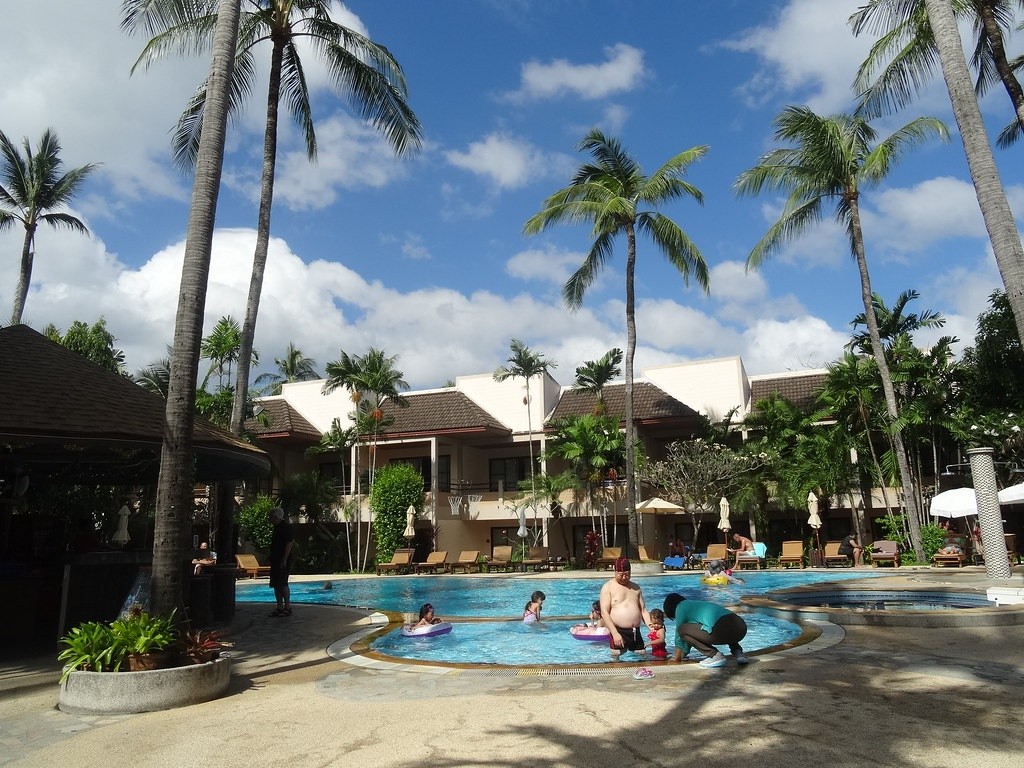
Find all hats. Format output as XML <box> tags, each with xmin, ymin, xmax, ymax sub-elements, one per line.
<box><xmin>614</xmin><ymin>556</ymin><xmax>631</xmax><ymax>572</ymax></box>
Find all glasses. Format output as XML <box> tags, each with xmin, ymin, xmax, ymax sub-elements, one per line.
<box><xmin>591</xmin><ymin>610</ymin><xmax>595</xmax><ymax>613</ymax></box>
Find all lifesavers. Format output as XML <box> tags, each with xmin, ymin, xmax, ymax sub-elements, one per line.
<box><xmin>701</xmin><ymin>576</ymin><xmax>729</xmax><ymax>586</ymax></box>
<box><xmin>401</xmin><ymin>622</ymin><xmax>453</xmax><ymax>637</ymax></box>
<box><xmin>569</xmin><ymin>622</ymin><xmax>611</xmax><ymax>641</ymax></box>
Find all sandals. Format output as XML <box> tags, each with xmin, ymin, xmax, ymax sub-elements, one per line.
<box><xmin>271</xmin><ymin>606</ymin><xmax>292</xmax><ymax>617</ymax></box>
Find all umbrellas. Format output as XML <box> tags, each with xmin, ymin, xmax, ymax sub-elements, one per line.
<box><xmin>930</xmin><ymin>487</ymin><xmax>982</xmax><ymax>546</ymax></box>
<box><xmin>626</xmin><ymin>496</ymin><xmax>684</xmax><ymax>560</ymax></box>
<box><xmin>517</xmin><ymin>505</ymin><xmax>529</xmax><ymax>562</ymax></box>
<box><xmin>402</xmin><ymin>504</ymin><xmax>418</xmax><ymax>574</ymax></box>
<box><xmin>112</xmin><ymin>504</ymin><xmax>132</xmax><ymax>550</ymax></box>
<box><xmin>717</xmin><ymin>496</ymin><xmax>732</xmax><ymax>566</ymax></box>
<box><xmin>807</xmin><ymin>491</ymin><xmax>824</xmax><ymax>559</ymax></box>
<box><xmin>998</xmin><ymin>482</ymin><xmax>1024</xmax><ymax>504</ymax></box>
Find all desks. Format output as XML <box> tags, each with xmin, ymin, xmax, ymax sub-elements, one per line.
<box><xmin>663</xmin><ymin>557</ymin><xmax>687</xmax><ymax>571</ymax></box>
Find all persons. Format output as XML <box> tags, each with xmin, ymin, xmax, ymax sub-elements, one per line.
<box><xmin>838</xmin><ymin>532</ymin><xmax>864</xmax><ymax>569</ymax></box>
<box><xmin>522</xmin><ymin>588</ymin><xmax>546</xmax><ymax>625</ymax></box>
<box><xmin>600</xmin><ymin>557</ymin><xmax>658</xmax><ymax>660</ymax></box>
<box><xmin>267</xmin><ymin>507</ymin><xmax>295</xmax><ymax>618</ymax></box>
<box><xmin>323</xmin><ymin>581</ymin><xmax>332</xmax><ymax>589</ymax></box>
<box><xmin>411</xmin><ymin>603</ymin><xmax>444</xmax><ymax>630</ymax></box>
<box><xmin>647</xmin><ymin>607</ymin><xmax>667</xmax><ymax>661</ymax></box>
<box><xmin>664</xmin><ymin>592</ymin><xmax>749</xmax><ymax>668</ymax></box>
<box><xmin>589</xmin><ymin>599</ymin><xmax>610</xmax><ymax>629</ymax></box>
<box><xmin>704</xmin><ymin>558</ymin><xmax>743</xmax><ymax>585</ymax></box>
<box><xmin>937</xmin><ymin>521</ymin><xmax>983</xmax><ymax>556</ymax></box>
<box><xmin>191</xmin><ymin>540</ymin><xmax>217</xmax><ymax>575</ymax></box>
<box><xmin>76</xmin><ymin>519</ymin><xmax>105</xmax><ymax>552</ymax></box>
<box><xmin>673</xmin><ymin>537</ymin><xmax>686</xmax><ymax>561</ymax></box>
<box><xmin>727</xmin><ymin>533</ymin><xmax>756</xmax><ymax>570</ymax></box>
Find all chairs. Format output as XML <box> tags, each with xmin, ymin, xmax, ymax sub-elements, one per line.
<box><xmin>824</xmin><ymin>541</ymin><xmax>851</xmax><ymax>567</ymax></box>
<box><xmin>637</xmin><ymin>545</ymin><xmax>683</xmax><ymax>561</ymax></box>
<box><xmin>871</xmin><ymin>541</ymin><xmax>901</xmax><ymax>568</ymax></box>
<box><xmin>235</xmin><ymin>554</ymin><xmax>270</xmax><ymax>580</ymax></box>
<box><xmin>703</xmin><ymin>544</ymin><xmax>728</xmax><ymax>569</ymax></box>
<box><xmin>779</xmin><ymin>541</ymin><xmax>807</xmax><ymax>569</ymax></box>
<box><xmin>934</xmin><ymin>534</ymin><xmax>1021</xmax><ymax>567</ymax></box>
<box><xmin>596</xmin><ymin>547</ymin><xmax>623</xmax><ymax>570</ymax></box>
<box><xmin>738</xmin><ymin>542</ymin><xmax>768</xmax><ymax>570</ymax></box>
<box><xmin>377</xmin><ymin>546</ymin><xmax>573</xmax><ymax>576</ymax></box>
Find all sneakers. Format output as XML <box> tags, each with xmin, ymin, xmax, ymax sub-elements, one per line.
<box><xmin>700</xmin><ymin>652</ymin><xmax>727</xmax><ymax>667</ymax></box>
<box><xmin>732</xmin><ymin>648</ymin><xmax>749</xmax><ymax>664</ymax></box>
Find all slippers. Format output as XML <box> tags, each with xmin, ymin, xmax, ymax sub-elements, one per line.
<box><xmin>634</xmin><ymin>668</ymin><xmax>654</xmax><ymax>680</ymax></box>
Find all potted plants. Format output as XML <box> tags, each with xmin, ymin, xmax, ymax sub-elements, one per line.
<box><xmin>57</xmin><ymin>607</ymin><xmax>223</xmax><ymax>684</ymax></box>
